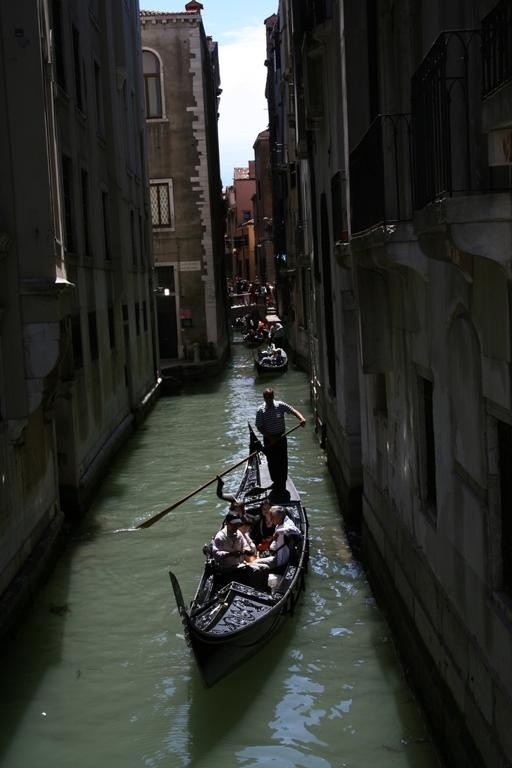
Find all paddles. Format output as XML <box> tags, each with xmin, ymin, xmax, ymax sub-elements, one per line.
<box><xmin>135</xmin><ymin>420</ymin><xmax>310</xmax><ymax>528</ymax></box>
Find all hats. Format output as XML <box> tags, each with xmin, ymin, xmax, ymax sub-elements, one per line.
<box><xmin>226</xmin><ymin>512</ymin><xmax>243</xmax><ymax>526</ymax></box>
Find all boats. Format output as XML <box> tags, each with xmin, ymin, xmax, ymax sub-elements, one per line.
<box><xmin>239</xmin><ymin>310</ymin><xmax>289</xmax><ymax>378</ymax></box>
<box><xmin>163</xmin><ymin>420</ymin><xmax>312</xmax><ymax>692</ymax></box>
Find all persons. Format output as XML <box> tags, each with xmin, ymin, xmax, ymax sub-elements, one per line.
<box><xmin>255</xmin><ymin>387</ymin><xmax>306</xmax><ymax>498</ymax></box>
<box><xmin>227</xmin><ymin>271</ymin><xmax>287</xmax><ymax>367</ymax></box>
<box><xmin>211</xmin><ymin>498</ymin><xmax>303</xmax><ymax>574</ymax></box>
